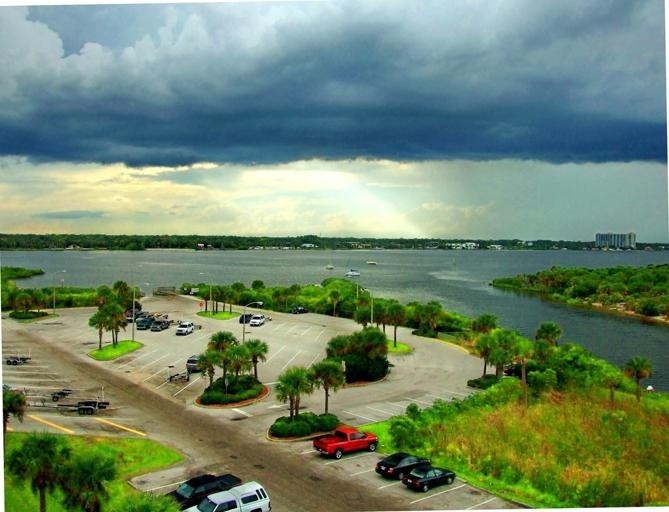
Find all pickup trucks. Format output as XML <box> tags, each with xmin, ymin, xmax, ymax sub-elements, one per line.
<box><xmin>313</xmin><ymin>427</ymin><xmax>378</xmax><ymax>459</ymax></box>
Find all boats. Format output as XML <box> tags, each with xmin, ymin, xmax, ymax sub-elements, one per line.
<box><xmin>326</xmin><ymin>261</ymin><xmax>376</xmax><ymax>276</ymax></box>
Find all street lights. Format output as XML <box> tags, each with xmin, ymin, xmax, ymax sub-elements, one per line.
<box><xmin>132</xmin><ymin>282</ymin><xmax>149</xmax><ymax>341</ymax></box>
<box><xmin>53</xmin><ymin>270</ymin><xmax>67</xmax><ymax>315</ymax></box>
<box><xmin>512</xmin><ymin>350</ymin><xmax>535</xmax><ymax>406</ymax></box>
<box><xmin>354</xmin><ymin>281</ymin><xmax>374</xmax><ymax>327</ymax></box>
<box><xmin>243</xmin><ymin>302</ymin><xmax>263</xmax><ymax>343</ymax></box>
<box><xmin>200</xmin><ymin>272</ymin><xmax>212</xmax><ymax>318</ymax></box>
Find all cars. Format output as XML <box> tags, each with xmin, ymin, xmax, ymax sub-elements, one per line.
<box><xmin>239</xmin><ymin>314</ymin><xmax>266</xmax><ymax>326</ymax></box>
<box><xmin>126</xmin><ymin>308</ymin><xmax>194</xmax><ymax>336</ymax></box>
<box><xmin>504</xmin><ymin>362</ymin><xmax>518</xmax><ymax>376</ymax></box>
<box><xmin>186</xmin><ymin>355</ymin><xmax>201</xmax><ymax>371</ymax></box>
<box><xmin>292</xmin><ymin>306</ymin><xmax>308</xmax><ymax>314</ymax></box>
<box><xmin>375</xmin><ymin>452</ymin><xmax>456</xmax><ymax>493</ymax></box>
<box><xmin>165</xmin><ymin>473</ymin><xmax>272</xmax><ymax>512</ymax></box>
